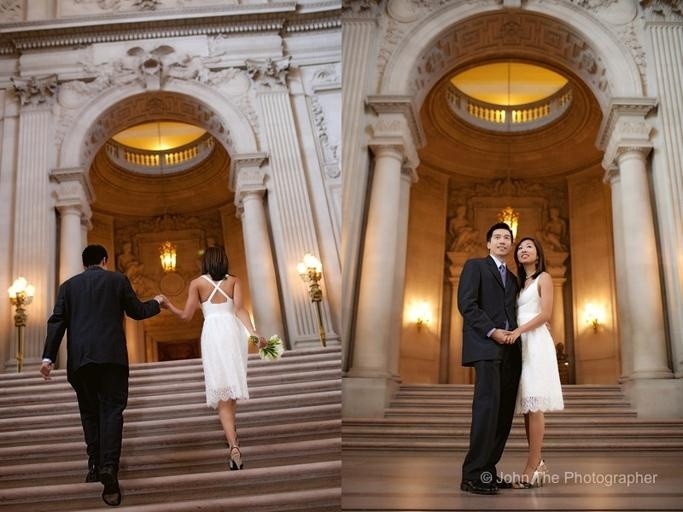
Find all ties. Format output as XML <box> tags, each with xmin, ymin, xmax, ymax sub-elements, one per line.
<box><xmin>500</xmin><ymin>264</ymin><xmax>506</xmax><ymax>287</ymax></box>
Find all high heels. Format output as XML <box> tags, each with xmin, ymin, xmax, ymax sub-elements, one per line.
<box><xmin>513</xmin><ymin>458</ymin><xmax>548</xmax><ymax>489</ymax></box>
<box><xmin>225</xmin><ymin>428</ymin><xmax>243</xmax><ymax>470</ymax></box>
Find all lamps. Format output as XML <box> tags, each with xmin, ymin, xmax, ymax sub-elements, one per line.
<box><xmin>583</xmin><ymin>302</ymin><xmax>604</xmax><ymax>333</ymax></box>
<box><xmin>498</xmin><ymin>63</ymin><xmax>519</xmax><ymax>243</ymax></box>
<box><xmin>158</xmin><ymin>121</ymin><xmax>177</xmax><ymax>274</ymax></box>
<box><xmin>411</xmin><ymin>301</ymin><xmax>433</xmax><ymax>335</ymax></box>
<box><xmin>6</xmin><ymin>277</ymin><xmax>36</xmax><ymax>373</ymax></box>
<box><xmin>298</xmin><ymin>251</ymin><xmax>334</xmax><ymax>347</ymax></box>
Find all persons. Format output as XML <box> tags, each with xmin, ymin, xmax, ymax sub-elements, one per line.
<box><xmin>160</xmin><ymin>245</ymin><xmax>268</xmax><ymax>471</ymax></box>
<box><xmin>502</xmin><ymin>236</ymin><xmax>564</xmax><ymax>489</ymax></box>
<box><xmin>457</xmin><ymin>223</ymin><xmax>551</xmax><ymax>493</ymax></box>
<box><xmin>535</xmin><ymin>207</ymin><xmax>567</xmax><ymax>249</ymax></box>
<box><xmin>40</xmin><ymin>244</ymin><xmax>165</xmax><ymax>508</ymax></box>
<box><xmin>449</xmin><ymin>204</ymin><xmax>472</xmax><ymax>252</ymax></box>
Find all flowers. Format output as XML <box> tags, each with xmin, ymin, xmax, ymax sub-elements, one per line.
<box><xmin>251</xmin><ymin>335</ymin><xmax>285</xmax><ymax>363</ymax></box>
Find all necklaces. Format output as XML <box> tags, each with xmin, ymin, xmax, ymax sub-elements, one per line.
<box><xmin>526</xmin><ymin>270</ymin><xmax>537</xmax><ymax>279</ymax></box>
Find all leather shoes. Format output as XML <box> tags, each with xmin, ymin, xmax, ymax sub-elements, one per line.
<box><xmin>460</xmin><ymin>478</ymin><xmax>498</xmax><ymax>495</ymax></box>
<box><xmin>86</xmin><ymin>462</ymin><xmax>98</xmax><ymax>482</ymax></box>
<box><xmin>494</xmin><ymin>474</ymin><xmax>512</xmax><ymax>488</ymax></box>
<box><xmin>99</xmin><ymin>467</ymin><xmax>121</xmax><ymax>505</ymax></box>
